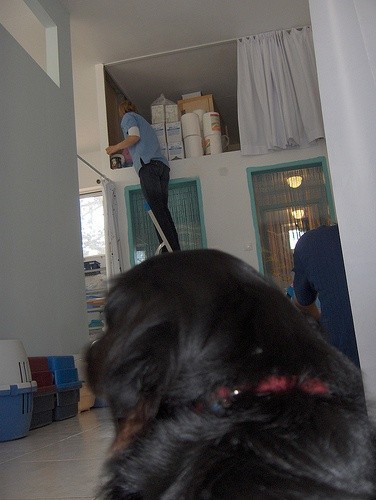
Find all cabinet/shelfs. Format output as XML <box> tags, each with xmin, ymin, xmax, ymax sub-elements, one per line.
<box><xmin>83</xmin><ymin>256</ymin><xmax>107</xmax><ymax>344</ymax></box>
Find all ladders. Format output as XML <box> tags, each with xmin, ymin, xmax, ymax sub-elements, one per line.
<box><xmin>144</xmin><ymin>200</ymin><xmax>174</xmax><ymax>256</ymax></box>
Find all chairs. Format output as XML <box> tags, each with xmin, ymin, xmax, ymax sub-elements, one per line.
<box><xmin>71</xmin><ymin>357</ymin><xmax>95</xmax><ymax>412</ymax></box>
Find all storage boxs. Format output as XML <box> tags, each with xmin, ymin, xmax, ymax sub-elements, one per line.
<box><xmin>165</xmin><ymin>121</ymin><xmax>182</xmax><ymax>142</ymax></box>
<box><xmin>168</xmin><ymin>142</ymin><xmax>185</xmax><ymax>159</ymax></box>
<box><xmin>165</xmin><ymin>105</ymin><xmax>177</xmax><ymax>122</ymax></box>
<box><xmin>151</xmin><ymin>124</ymin><xmax>165</xmax><ymax>143</ymax></box>
<box><xmin>44</xmin><ymin>356</ymin><xmax>84</xmax><ymax>419</ymax></box>
<box><xmin>159</xmin><ymin>144</ymin><xmax>169</xmax><ymax>161</ymax></box>
<box><xmin>26</xmin><ymin>357</ymin><xmax>56</xmax><ymax>430</ymax></box>
<box><xmin>0</xmin><ymin>340</ymin><xmax>38</xmax><ymax>442</ymax></box>
<box><xmin>151</xmin><ymin>105</ymin><xmax>164</xmax><ymax>124</ymax></box>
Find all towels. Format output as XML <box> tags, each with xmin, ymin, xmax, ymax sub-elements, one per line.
<box><xmin>84</xmin><ymin>274</ymin><xmax>107</xmax><ymax>289</ymax></box>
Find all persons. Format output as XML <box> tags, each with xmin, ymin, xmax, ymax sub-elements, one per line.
<box><xmin>105</xmin><ymin>101</ymin><xmax>181</xmax><ymax>255</ymax></box>
<box><xmin>293</xmin><ymin>224</ymin><xmax>361</xmax><ymax>369</ymax></box>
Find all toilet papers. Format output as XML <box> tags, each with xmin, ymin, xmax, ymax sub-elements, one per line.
<box><xmin>203</xmin><ymin>134</ymin><xmax>223</xmax><ymax>155</ymax></box>
<box><xmin>191</xmin><ymin>109</ymin><xmax>207</xmax><ymax>131</ymax></box>
<box><xmin>183</xmin><ymin>135</ymin><xmax>203</xmax><ymax>159</ymax></box>
<box><xmin>203</xmin><ymin>112</ymin><xmax>221</xmax><ymax>138</ymax></box>
<box><xmin>180</xmin><ymin>112</ymin><xmax>200</xmax><ymax>138</ymax></box>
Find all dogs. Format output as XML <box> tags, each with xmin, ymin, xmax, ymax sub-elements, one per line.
<box><xmin>81</xmin><ymin>249</ymin><xmax>376</xmax><ymax>500</ymax></box>
